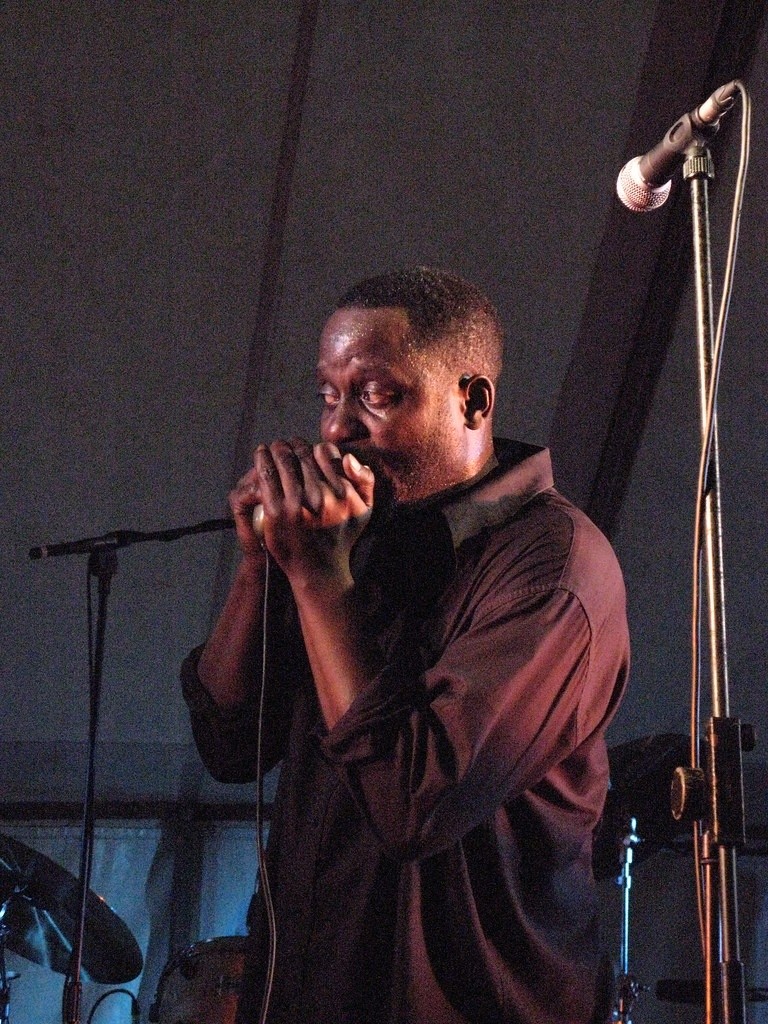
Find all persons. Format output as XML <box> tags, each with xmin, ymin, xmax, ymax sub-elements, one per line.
<box><xmin>182</xmin><ymin>266</ymin><xmax>629</xmax><ymax>1023</ymax></box>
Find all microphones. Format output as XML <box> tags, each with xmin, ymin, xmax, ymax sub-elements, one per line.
<box><xmin>617</xmin><ymin>79</ymin><xmax>741</xmax><ymax>213</ymax></box>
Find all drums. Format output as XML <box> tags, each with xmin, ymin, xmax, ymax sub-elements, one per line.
<box><xmin>155</xmin><ymin>937</ymin><xmax>244</xmax><ymax>1024</ymax></box>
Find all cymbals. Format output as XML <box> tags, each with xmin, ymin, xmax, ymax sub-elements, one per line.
<box><xmin>0</xmin><ymin>834</ymin><xmax>143</xmax><ymax>984</ymax></box>
<box><xmin>586</xmin><ymin>734</ymin><xmax>705</xmax><ymax>881</ymax></box>
